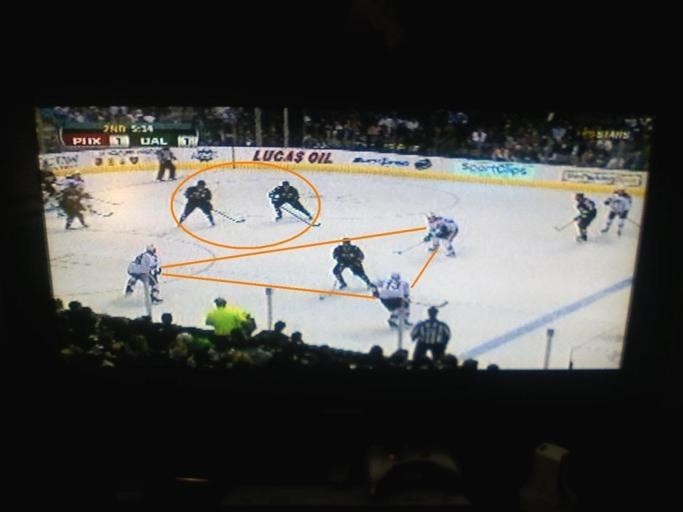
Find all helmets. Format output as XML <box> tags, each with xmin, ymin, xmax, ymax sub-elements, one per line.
<box><xmin>147</xmin><ymin>244</ymin><xmax>155</xmax><ymax>254</ymax></box>
<box><xmin>426</xmin><ymin>212</ymin><xmax>435</xmax><ymax>219</ymax></box>
<box><xmin>391</xmin><ymin>272</ymin><xmax>400</xmax><ymax>280</ymax></box>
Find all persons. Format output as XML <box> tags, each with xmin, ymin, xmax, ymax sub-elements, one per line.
<box><xmin>36</xmin><ymin>106</ymin><xmax>654</xmax><ymax>373</ymax></box>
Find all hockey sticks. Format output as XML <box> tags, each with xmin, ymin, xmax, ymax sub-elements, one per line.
<box><xmin>555</xmin><ymin>215</ymin><xmax>583</xmax><ymax>232</ymax></box>
<box><xmin>211</xmin><ymin>208</ymin><xmax>246</xmax><ymax>223</ymax></box>
<box><xmin>281</xmin><ymin>206</ymin><xmax>321</xmax><ymax>227</ymax></box>
<box><xmin>89</xmin><ymin>209</ymin><xmax>114</xmax><ymax>217</ymax></box>
<box><xmin>411</xmin><ymin>300</ymin><xmax>449</xmax><ymax>308</ymax></box>
